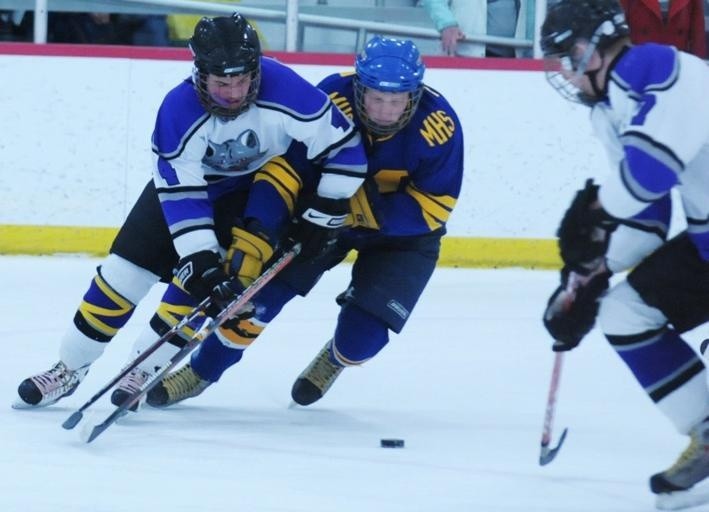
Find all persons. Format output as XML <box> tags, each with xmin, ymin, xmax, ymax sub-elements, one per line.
<box><xmin>147</xmin><ymin>38</ymin><xmax>467</xmax><ymax>403</ymax></box>
<box><xmin>17</xmin><ymin>14</ymin><xmax>369</xmax><ymax>427</ymax></box>
<box><xmin>539</xmin><ymin>1</ymin><xmax>709</xmax><ymax>501</ymax></box>
<box><xmin>427</xmin><ymin>0</ymin><xmax>528</xmax><ymax>56</ymax></box>
<box><xmin>614</xmin><ymin>0</ymin><xmax>709</xmax><ymax>60</ymax></box>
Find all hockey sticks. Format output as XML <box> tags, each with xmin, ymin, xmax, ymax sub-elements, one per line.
<box><xmin>540</xmin><ymin>177</ymin><xmax>595</xmax><ymax>465</ymax></box>
<box><xmin>87</xmin><ymin>242</ymin><xmax>304</xmax><ymax>444</ymax></box>
<box><xmin>62</xmin><ymin>296</ymin><xmax>213</xmax><ymax>429</ymax></box>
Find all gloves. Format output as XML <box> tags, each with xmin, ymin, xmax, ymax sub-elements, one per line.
<box><xmin>543</xmin><ymin>267</ymin><xmax>611</xmax><ymax>350</ymax></box>
<box><xmin>560</xmin><ymin>179</ymin><xmax>619</xmax><ymax>274</ymax></box>
<box><xmin>280</xmin><ymin>190</ymin><xmax>351</xmax><ymax>263</ymax></box>
<box><xmin>173</xmin><ymin>250</ymin><xmax>254</xmax><ymax>326</ymax></box>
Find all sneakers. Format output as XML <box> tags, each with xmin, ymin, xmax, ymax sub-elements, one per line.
<box><xmin>18</xmin><ymin>362</ymin><xmax>88</xmax><ymax>406</ymax></box>
<box><xmin>146</xmin><ymin>361</ymin><xmax>210</xmax><ymax>406</ymax></box>
<box><xmin>291</xmin><ymin>338</ymin><xmax>345</xmax><ymax>404</ymax></box>
<box><xmin>111</xmin><ymin>363</ymin><xmax>150</xmax><ymax>412</ymax></box>
<box><xmin>649</xmin><ymin>418</ymin><xmax>709</xmax><ymax>494</ymax></box>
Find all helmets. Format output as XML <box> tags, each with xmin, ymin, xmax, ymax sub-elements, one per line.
<box><xmin>541</xmin><ymin>0</ymin><xmax>627</xmax><ymax>104</ymax></box>
<box><xmin>354</xmin><ymin>35</ymin><xmax>426</xmax><ymax>134</ymax></box>
<box><xmin>189</xmin><ymin>13</ymin><xmax>262</xmax><ymax>116</ymax></box>
